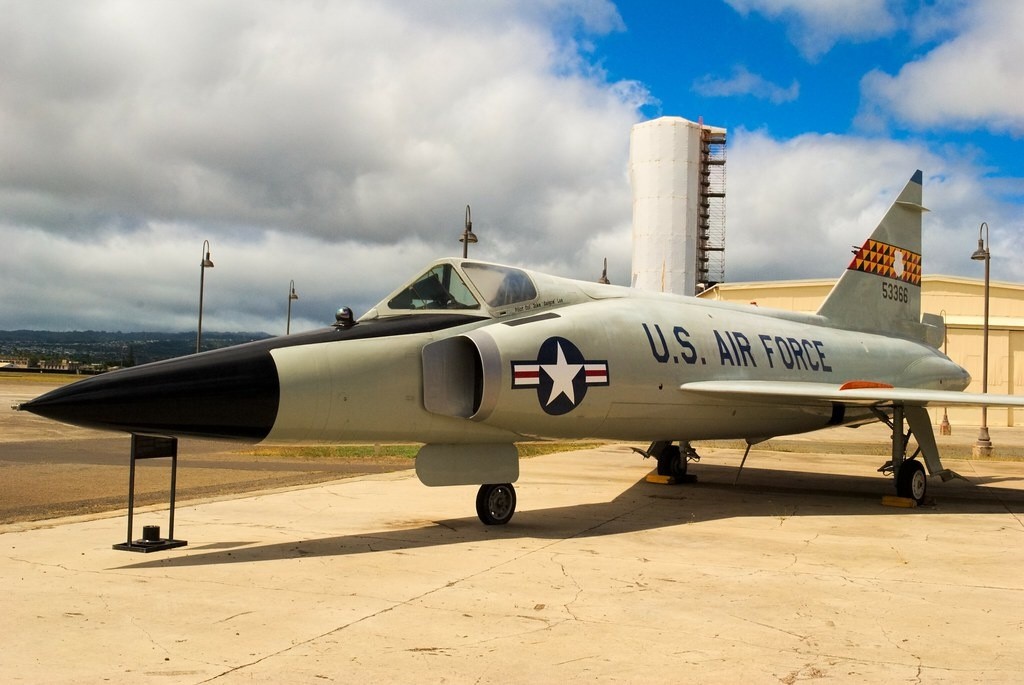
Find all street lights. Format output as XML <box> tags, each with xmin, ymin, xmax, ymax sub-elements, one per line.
<box><xmin>458</xmin><ymin>200</ymin><xmax>478</xmax><ymax>262</ymax></box>
<box><xmin>196</xmin><ymin>240</ymin><xmax>215</xmax><ymax>355</ymax></box>
<box><xmin>971</xmin><ymin>221</ymin><xmax>994</xmax><ymax>449</ymax></box>
<box><xmin>287</xmin><ymin>280</ymin><xmax>298</xmax><ymax>335</ymax></box>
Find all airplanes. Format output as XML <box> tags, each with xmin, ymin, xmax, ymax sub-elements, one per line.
<box><xmin>12</xmin><ymin>171</ymin><xmax>1023</xmax><ymax>524</ymax></box>
